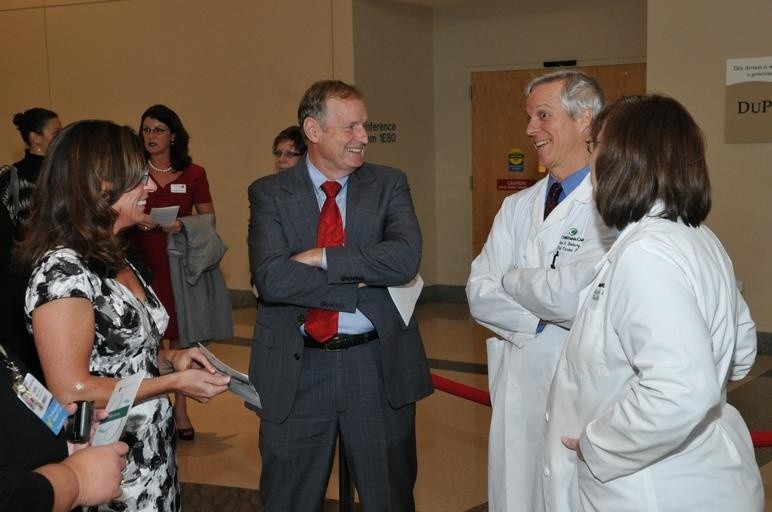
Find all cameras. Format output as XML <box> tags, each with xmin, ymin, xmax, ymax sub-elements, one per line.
<box><xmin>66</xmin><ymin>400</ymin><xmax>95</xmax><ymax>443</ymax></box>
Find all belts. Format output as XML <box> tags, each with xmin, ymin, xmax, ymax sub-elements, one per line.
<box><xmin>303</xmin><ymin>330</ymin><xmax>378</xmax><ymax>352</ymax></box>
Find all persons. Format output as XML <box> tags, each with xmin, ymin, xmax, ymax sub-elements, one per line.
<box><xmin>0</xmin><ymin>337</ymin><xmax>130</xmax><ymax>512</ymax></box>
<box><xmin>126</xmin><ymin>104</ymin><xmax>216</xmax><ymax>442</ymax></box>
<box><xmin>244</xmin><ymin>79</ymin><xmax>435</xmax><ymax>511</ymax></box>
<box><xmin>533</xmin><ymin>94</ymin><xmax>764</xmax><ymax>512</ymax></box>
<box><xmin>464</xmin><ymin>70</ymin><xmax>618</xmax><ymax>511</ymax></box>
<box><xmin>272</xmin><ymin>126</ymin><xmax>308</xmax><ymax>173</ymax></box>
<box><xmin>23</xmin><ymin>119</ymin><xmax>231</xmax><ymax>512</ymax></box>
<box><xmin>0</xmin><ymin>107</ymin><xmax>63</xmax><ymax>240</ymax></box>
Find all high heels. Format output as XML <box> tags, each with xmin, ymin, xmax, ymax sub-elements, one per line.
<box><xmin>172</xmin><ymin>405</ymin><xmax>195</xmax><ymax>440</ymax></box>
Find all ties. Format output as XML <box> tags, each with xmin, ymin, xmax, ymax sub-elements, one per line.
<box><xmin>543</xmin><ymin>182</ymin><xmax>562</xmax><ymax>221</ymax></box>
<box><xmin>304</xmin><ymin>181</ymin><xmax>344</xmax><ymax>344</ymax></box>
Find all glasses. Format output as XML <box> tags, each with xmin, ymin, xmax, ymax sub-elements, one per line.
<box><xmin>142</xmin><ymin>127</ymin><xmax>169</xmax><ymax>136</ymax></box>
<box><xmin>142</xmin><ymin>164</ymin><xmax>150</xmax><ymax>185</ymax></box>
<box><xmin>585</xmin><ymin>139</ymin><xmax>604</xmax><ymax>155</ymax></box>
<box><xmin>274</xmin><ymin>150</ymin><xmax>303</xmax><ymax>158</ymax></box>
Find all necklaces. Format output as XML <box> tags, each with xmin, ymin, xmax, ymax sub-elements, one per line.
<box><xmin>147</xmin><ymin>159</ymin><xmax>172</xmax><ymax>173</ymax></box>
